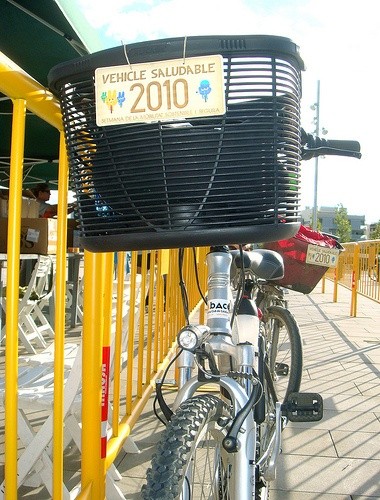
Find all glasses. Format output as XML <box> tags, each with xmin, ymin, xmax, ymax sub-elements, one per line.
<box><xmin>40</xmin><ymin>190</ymin><xmax>50</xmax><ymax>194</ymax></box>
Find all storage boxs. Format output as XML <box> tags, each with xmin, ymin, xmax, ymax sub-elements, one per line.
<box><xmin>0</xmin><ymin>199</ymin><xmax>80</xmax><ymax>255</ymax></box>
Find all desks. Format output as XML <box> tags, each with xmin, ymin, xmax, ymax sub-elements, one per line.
<box><xmin>0</xmin><ymin>253</ymin><xmax>83</xmax><ymax>328</ymax></box>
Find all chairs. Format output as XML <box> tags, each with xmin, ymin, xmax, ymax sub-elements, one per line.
<box><xmin>0</xmin><ymin>255</ymin><xmax>149</xmax><ymax>500</ymax></box>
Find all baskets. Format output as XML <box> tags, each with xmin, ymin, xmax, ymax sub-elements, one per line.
<box><xmin>262</xmin><ymin>224</ymin><xmax>345</xmax><ymax>295</ymax></box>
<box><xmin>47</xmin><ymin>35</ymin><xmax>306</xmax><ymax>252</ymax></box>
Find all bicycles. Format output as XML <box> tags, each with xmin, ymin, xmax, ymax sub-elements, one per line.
<box><xmin>369</xmin><ymin>261</ymin><xmax>380</xmax><ymax>282</ymax></box>
<box><xmin>253</xmin><ymin>220</ymin><xmax>345</xmax><ymax>409</ymax></box>
<box><xmin>46</xmin><ymin>35</ymin><xmax>363</xmax><ymax>500</ymax></box>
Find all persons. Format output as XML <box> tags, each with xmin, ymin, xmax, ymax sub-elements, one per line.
<box><xmin>111</xmin><ymin>241</ymin><xmax>257</xmax><ymax>314</ymax></box>
<box><xmin>19</xmin><ymin>183</ymin><xmax>79</xmax><ymax>314</ymax></box>
